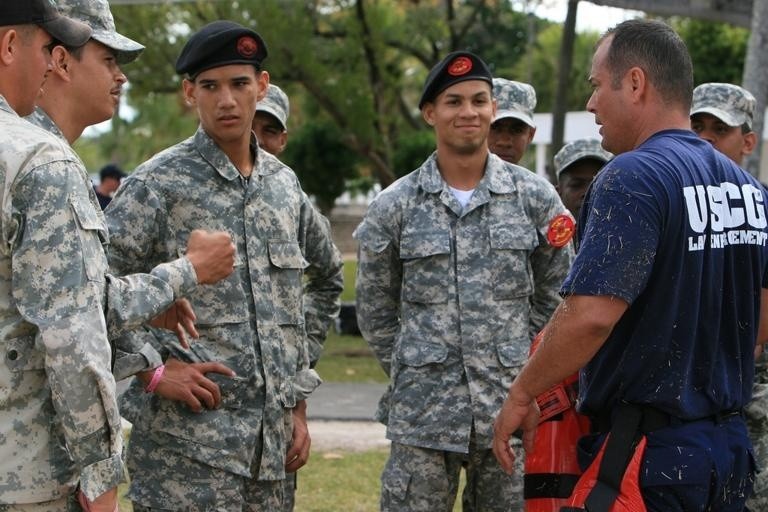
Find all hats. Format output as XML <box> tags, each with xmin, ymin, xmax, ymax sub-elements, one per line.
<box><xmin>174</xmin><ymin>20</ymin><xmax>269</xmax><ymax>81</ymax></box>
<box><xmin>553</xmin><ymin>137</ymin><xmax>613</xmax><ymax>184</ymax></box>
<box><xmin>690</xmin><ymin>81</ymin><xmax>757</xmax><ymax>130</ymax></box>
<box><xmin>418</xmin><ymin>50</ymin><xmax>492</xmax><ymax>109</ymax></box>
<box><xmin>490</xmin><ymin>78</ymin><xmax>538</xmax><ymax>130</ymax></box>
<box><xmin>0</xmin><ymin>0</ymin><xmax>93</xmax><ymax>49</ymax></box>
<box><xmin>99</xmin><ymin>164</ymin><xmax>127</xmax><ymax>179</ymax></box>
<box><xmin>253</xmin><ymin>84</ymin><xmax>290</xmax><ymax>132</ymax></box>
<box><xmin>51</xmin><ymin>1</ymin><xmax>147</xmax><ymax>64</ymax></box>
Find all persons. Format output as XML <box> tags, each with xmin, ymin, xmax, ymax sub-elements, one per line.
<box><xmin>350</xmin><ymin>49</ymin><xmax>580</xmax><ymax>512</ymax></box>
<box><xmin>248</xmin><ymin>81</ymin><xmax>292</xmax><ymax>159</ymax></box>
<box><xmin>487</xmin><ymin>77</ymin><xmax>541</xmax><ymax>168</ymax></box>
<box><xmin>552</xmin><ymin>137</ymin><xmax>619</xmax><ymax>214</ymax></box>
<box><xmin>89</xmin><ymin>161</ymin><xmax>128</xmax><ymax>211</ymax></box>
<box><xmin>688</xmin><ymin>79</ymin><xmax>768</xmax><ymax>511</ymax></box>
<box><xmin>97</xmin><ymin>17</ymin><xmax>344</xmax><ymax>512</ymax></box>
<box><xmin>24</xmin><ymin>0</ymin><xmax>238</xmax><ymax>512</ymax></box>
<box><xmin>491</xmin><ymin>15</ymin><xmax>767</xmax><ymax>510</ymax></box>
<box><xmin>0</xmin><ymin>0</ymin><xmax>128</xmax><ymax>512</ymax></box>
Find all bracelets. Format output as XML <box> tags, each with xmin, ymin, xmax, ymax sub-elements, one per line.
<box><xmin>145</xmin><ymin>362</ymin><xmax>165</xmax><ymax>393</ymax></box>
